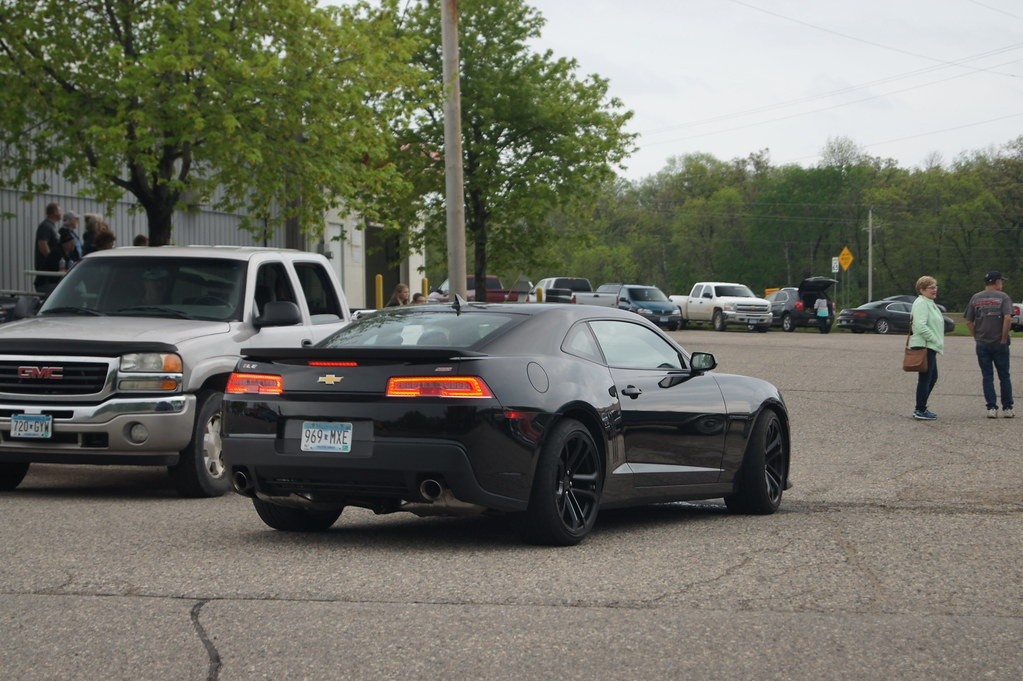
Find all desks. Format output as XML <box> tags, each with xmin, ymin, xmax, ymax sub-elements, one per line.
<box><xmin>24</xmin><ymin>270</ymin><xmax>67</xmax><ymax>277</ymax></box>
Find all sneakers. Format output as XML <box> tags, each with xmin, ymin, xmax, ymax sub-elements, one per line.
<box><xmin>987</xmin><ymin>409</ymin><xmax>999</xmax><ymax>418</ymax></box>
<box><xmin>1003</xmin><ymin>408</ymin><xmax>1015</xmax><ymax>418</ymax></box>
<box><xmin>913</xmin><ymin>409</ymin><xmax>937</xmax><ymax>419</ymax></box>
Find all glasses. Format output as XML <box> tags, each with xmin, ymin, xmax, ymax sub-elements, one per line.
<box><xmin>925</xmin><ymin>284</ymin><xmax>937</xmax><ymax>290</ymax></box>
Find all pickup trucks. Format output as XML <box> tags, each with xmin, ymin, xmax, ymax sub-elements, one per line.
<box><xmin>0</xmin><ymin>244</ymin><xmax>425</xmax><ymax>498</ymax></box>
<box><xmin>668</xmin><ymin>281</ymin><xmax>773</xmax><ymax>332</ymax></box>
<box><xmin>524</xmin><ymin>277</ymin><xmax>619</xmax><ymax>308</ymax></box>
<box><xmin>429</xmin><ymin>275</ymin><xmax>521</xmax><ymax>304</ymax></box>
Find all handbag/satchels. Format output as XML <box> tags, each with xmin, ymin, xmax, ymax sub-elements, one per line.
<box><xmin>903</xmin><ymin>348</ymin><xmax>928</xmax><ymax>372</ymax></box>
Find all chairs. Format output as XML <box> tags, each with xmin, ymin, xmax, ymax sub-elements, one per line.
<box><xmin>416</xmin><ymin>330</ymin><xmax>448</xmax><ymax>346</ymax></box>
<box><xmin>255</xmin><ymin>286</ymin><xmax>275</xmax><ymax>313</ymax></box>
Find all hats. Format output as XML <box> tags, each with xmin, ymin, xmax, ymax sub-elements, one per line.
<box><xmin>62</xmin><ymin>211</ymin><xmax>80</xmax><ymax>221</ymax></box>
<box><xmin>58</xmin><ymin>227</ymin><xmax>74</xmax><ymax>243</ymax></box>
<box><xmin>985</xmin><ymin>271</ymin><xmax>1008</xmax><ymax>281</ymax></box>
<box><xmin>144</xmin><ymin>265</ymin><xmax>167</xmax><ymax>280</ymax></box>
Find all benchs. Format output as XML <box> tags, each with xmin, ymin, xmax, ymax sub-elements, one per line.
<box><xmin>0</xmin><ymin>289</ymin><xmax>98</xmax><ymax>300</ymax></box>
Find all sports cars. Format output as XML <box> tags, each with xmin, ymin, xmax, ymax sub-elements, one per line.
<box><xmin>220</xmin><ymin>294</ymin><xmax>792</xmax><ymax>547</ymax></box>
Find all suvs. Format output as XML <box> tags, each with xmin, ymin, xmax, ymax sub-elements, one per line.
<box><xmin>594</xmin><ymin>282</ymin><xmax>682</xmax><ymax>332</ymax></box>
<box><xmin>757</xmin><ymin>277</ymin><xmax>840</xmax><ymax>333</ymax></box>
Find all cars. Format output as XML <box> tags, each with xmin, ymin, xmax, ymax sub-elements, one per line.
<box><xmin>878</xmin><ymin>294</ymin><xmax>946</xmax><ymax>314</ymax></box>
<box><xmin>836</xmin><ymin>301</ymin><xmax>957</xmax><ymax>335</ymax></box>
<box><xmin>1008</xmin><ymin>302</ymin><xmax>1023</xmax><ymax>332</ymax></box>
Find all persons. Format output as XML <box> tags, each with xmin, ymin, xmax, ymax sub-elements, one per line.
<box><xmin>386</xmin><ymin>283</ymin><xmax>411</xmax><ymax>307</ymax></box>
<box><xmin>413</xmin><ymin>293</ymin><xmax>427</xmax><ymax>304</ymax></box>
<box><xmin>33</xmin><ymin>202</ymin><xmax>149</xmax><ymax>301</ymax></box>
<box><xmin>814</xmin><ymin>293</ymin><xmax>829</xmax><ymax>333</ymax></box>
<box><xmin>963</xmin><ymin>269</ymin><xmax>1016</xmax><ymax>419</ymax></box>
<box><xmin>907</xmin><ymin>274</ymin><xmax>944</xmax><ymax>421</ymax></box>
